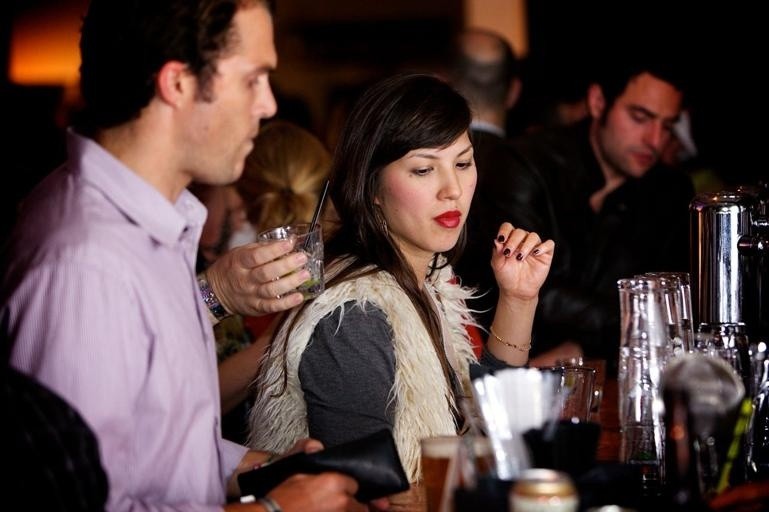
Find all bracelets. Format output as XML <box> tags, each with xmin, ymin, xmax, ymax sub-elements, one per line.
<box><xmin>255</xmin><ymin>494</ymin><xmax>284</xmax><ymax>512</ymax></box>
<box><xmin>196</xmin><ymin>271</ymin><xmax>234</xmax><ymax>326</ymax></box>
<box><xmin>488</xmin><ymin>325</ymin><xmax>532</xmax><ymax>355</ymax></box>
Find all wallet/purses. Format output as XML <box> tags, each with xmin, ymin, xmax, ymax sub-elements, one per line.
<box><xmin>236</xmin><ymin>429</ymin><xmax>412</xmax><ymax>506</ymax></box>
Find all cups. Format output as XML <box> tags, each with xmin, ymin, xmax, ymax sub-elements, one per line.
<box><xmin>550</xmin><ymin>366</ymin><xmax>597</xmax><ymax>426</ymax></box>
<box><xmin>616</xmin><ymin>274</ymin><xmax>769</xmax><ymax>464</ymax></box>
<box><xmin>417</xmin><ymin>434</ymin><xmax>502</xmax><ymax>512</ymax></box>
<box><xmin>255</xmin><ymin>223</ymin><xmax>324</xmax><ymax>300</ymax></box>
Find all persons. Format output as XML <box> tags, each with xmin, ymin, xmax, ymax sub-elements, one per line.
<box><xmin>662</xmin><ymin>113</ymin><xmax>698</xmax><ymax>175</ymax></box>
<box><xmin>248</xmin><ymin>72</ymin><xmax>553</xmax><ymax>487</ymax></box>
<box><xmin>5</xmin><ymin>3</ymin><xmax>394</xmax><ymax>510</ymax></box>
<box><xmin>425</xmin><ymin>28</ymin><xmax>555</xmax><ymax>237</ymax></box>
<box><xmin>194</xmin><ymin>234</ymin><xmax>310</xmax><ymax>325</ymax></box>
<box><xmin>320</xmin><ymin>84</ymin><xmax>363</xmax><ymax>146</ymax></box>
<box><xmin>490</xmin><ymin>40</ymin><xmax>725</xmax><ymax>377</ymax></box>
<box><xmin>0</xmin><ymin>364</ymin><xmax>106</xmax><ymax>512</ymax></box>
<box><xmin>237</xmin><ymin>123</ymin><xmax>339</xmax><ymax>241</ymax></box>
<box><xmin>193</xmin><ymin>184</ymin><xmax>287</xmax><ymax>416</ymax></box>
<box><xmin>525</xmin><ymin>77</ymin><xmax>590</xmax><ymax>136</ymax></box>
